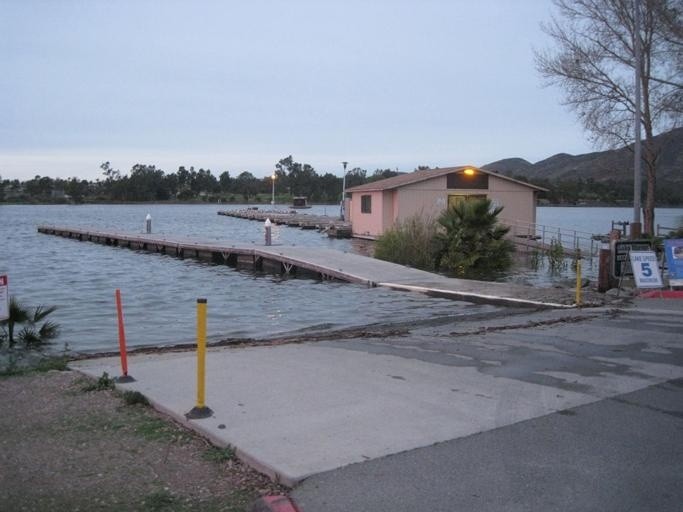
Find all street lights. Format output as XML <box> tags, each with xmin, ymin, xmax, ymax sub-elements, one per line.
<box><xmin>339</xmin><ymin>160</ymin><xmax>349</xmax><ymax>218</ymax></box>
<box><xmin>270</xmin><ymin>174</ymin><xmax>276</xmax><ymax>205</ymax></box>
